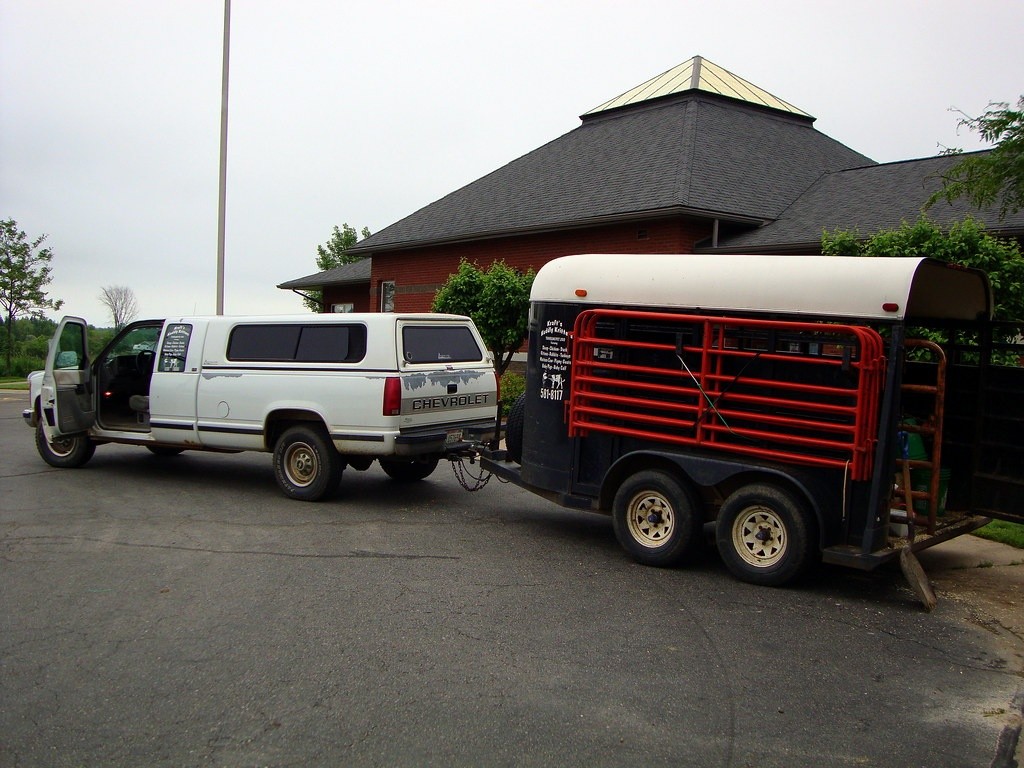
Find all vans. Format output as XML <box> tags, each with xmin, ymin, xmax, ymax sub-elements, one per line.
<box><xmin>22</xmin><ymin>313</ymin><xmax>504</xmax><ymax>503</ymax></box>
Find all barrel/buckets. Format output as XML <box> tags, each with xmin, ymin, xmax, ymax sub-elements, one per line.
<box><xmin>913</xmin><ymin>468</ymin><xmax>951</xmax><ymax>517</ymax></box>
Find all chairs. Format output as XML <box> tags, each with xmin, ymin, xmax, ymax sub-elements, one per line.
<box><xmin>129</xmin><ymin>395</ymin><xmax>149</xmax><ymax>425</ymax></box>
